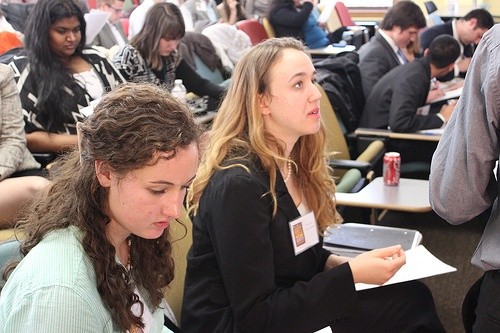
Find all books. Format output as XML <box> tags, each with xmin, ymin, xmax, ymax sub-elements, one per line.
<box><xmin>354</xmin><ymin>245</ymin><xmax>456</xmax><ymax>292</ymax></box>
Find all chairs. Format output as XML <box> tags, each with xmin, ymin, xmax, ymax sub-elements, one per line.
<box><xmin>0</xmin><ymin>0</ymin><xmax>443</xmax><ymax>326</ymax></box>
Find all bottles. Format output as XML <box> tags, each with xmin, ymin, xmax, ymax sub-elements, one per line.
<box><xmin>171</xmin><ymin>79</ymin><xmax>187</xmax><ymax>103</ymax></box>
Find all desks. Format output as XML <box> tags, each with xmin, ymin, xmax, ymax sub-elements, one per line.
<box><xmin>331</xmin><ymin>175</ymin><xmax>433</xmax><ymax>213</ymax></box>
<box><xmin>355</xmin><ymin>123</ymin><xmax>446</xmax><ymax>142</ymax></box>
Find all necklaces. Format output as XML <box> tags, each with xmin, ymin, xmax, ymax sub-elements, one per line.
<box><xmin>284</xmin><ymin>156</ymin><xmax>292</xmax><ymax>182</ymax></box>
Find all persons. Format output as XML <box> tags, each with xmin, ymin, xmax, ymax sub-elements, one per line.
<box><xmin>429</xmin><ymin>23</ymin><xmax>500</xmax><ymax>333</ymax></box>
<box><xmin>0</xmin><ymin>0</ymin><xmax>348</xmax><ymax>228</ymax></box>
<box><xmin>182</xmin><ymin>34</ymin><xmax>446</xmax><ymax>333</ymax></box>
<box><xmin>356</xmin><ymin>0</ymin><xmax>445</xmax><ymax>106</ymax></box>
<box><xmin>415</xmin><ymin>8</ymin><xmax>494</xmax><ymax>82</ymax></box>
<box><xmin>0</xmin><ymin>83</ymin><xmax>212</xmax><ymax>333</ymax></box>
<box><xmin>356</xmin><ymin>34</ymin><xmax>461</xmax><ymax>170</ymax></box>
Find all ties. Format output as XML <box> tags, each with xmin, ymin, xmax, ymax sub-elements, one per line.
<box><xmin>397</xmin><ymin>48</ymin><xmax>408</xmax><ymax>64</ymax></box>
